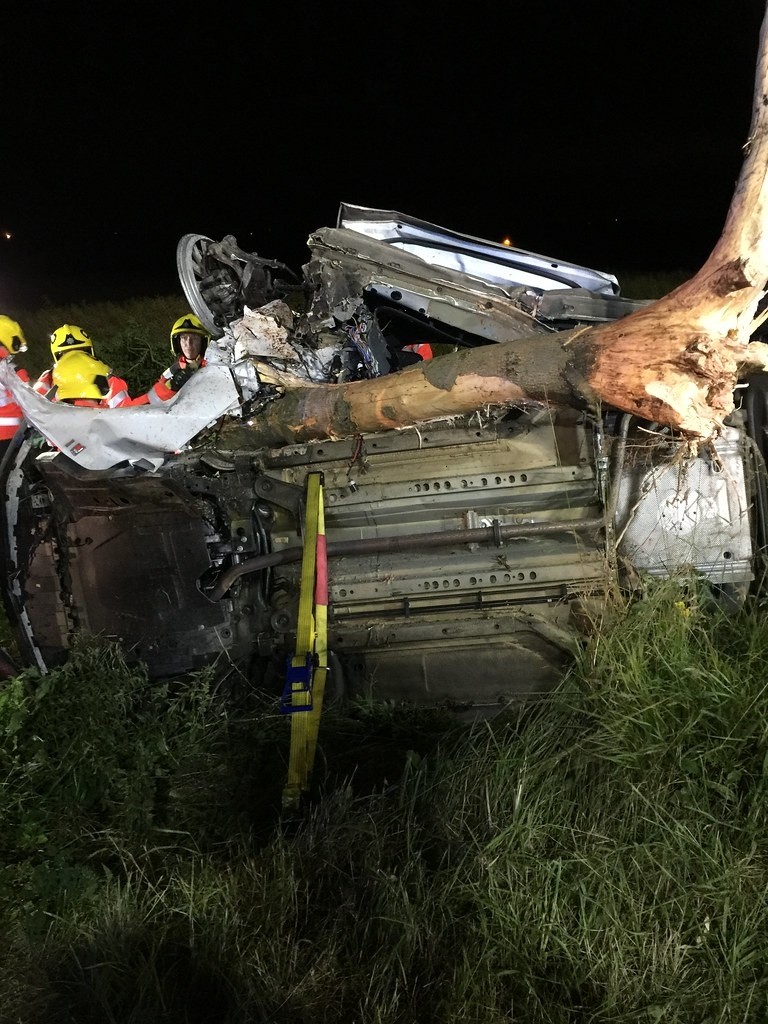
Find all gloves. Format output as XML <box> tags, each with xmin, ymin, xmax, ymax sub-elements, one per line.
<box><xmin>170</xmin><ymin>369</ymin><xmax>191</xmax><ymax>391</ymax></box>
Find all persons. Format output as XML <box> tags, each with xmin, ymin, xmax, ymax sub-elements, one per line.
<box><xmin>0</xmin><ymin>314</ymin><xmax>131</xmax><ymax>467</ymax></box>
<box><xmin>132</xmin><ymin>314</ymin><xmax>209</xmax><ymax>404</ymax></box>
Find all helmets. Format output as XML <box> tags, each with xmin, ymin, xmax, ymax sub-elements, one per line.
<box><xmin>52</xmin><ymin>351</ymin><xmax>115</xmax><ymax>400</ymax></box>
<box><xmin>169</xmin><ymin>314</ymin><xmax>210</xmax><ymax>356</ymax></box>
<box><xmin>0</xmin><ymin>314</ymin><xmax>28</xmax><ymax>354</ymax></box>
<box><xmin>50</xmin><ymin>323</ymin><xmax>95</xmax><ymax>362</ymax></box>
